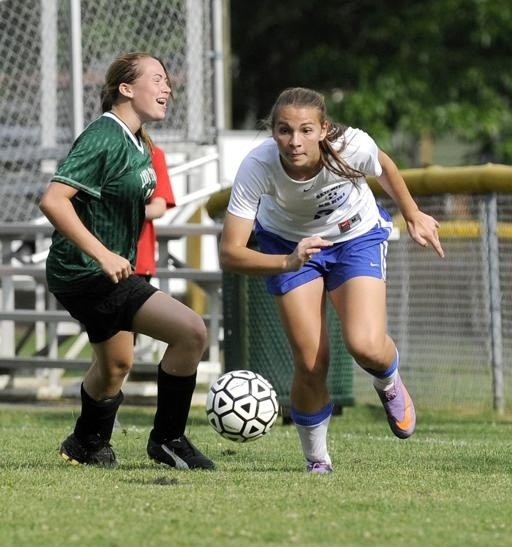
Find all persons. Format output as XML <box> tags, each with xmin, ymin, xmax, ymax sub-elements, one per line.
<box><xmin>98</xmin><ymin>83</ymin><xmax>177</xmax><ymax>432</ymax></box>
<box><xmin>39</xmin><ymin>52</ymin><xmax>217</xmax><ymax>472</ymax></box>
<box><xmin>218</xmin><ymin>84</ymin><xmax>446</xmax><ymax>474</ymax></box>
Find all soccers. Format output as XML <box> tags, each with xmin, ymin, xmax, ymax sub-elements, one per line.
<box><xmin>205</xmin><ymin>370</ymin><xmax>279</xmax><ymax>443</ymax></box>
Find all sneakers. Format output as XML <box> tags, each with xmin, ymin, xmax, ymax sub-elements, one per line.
<box><xmin>59</xmin><ymin>431</ymin><xmax>120</xmax><ymax>470</ymax></box>
<box><xmin>112</xmin><ymin>412</ymin><xmax>124</xmax><ymax>435</ymax></box>
<box><xmin>372</xmin><ymin>370</ymin><xmax>417</xmax><ymax>439</ymax></box>
<box><xmin>305</xmin><ymin>458</ymin><xmax>333</xmax><ymax>478</ymax></box>
<box><xmin>143</xmin><ymin>430</ymin><xmax>215</xmax><ymax>471</ymax></box>
